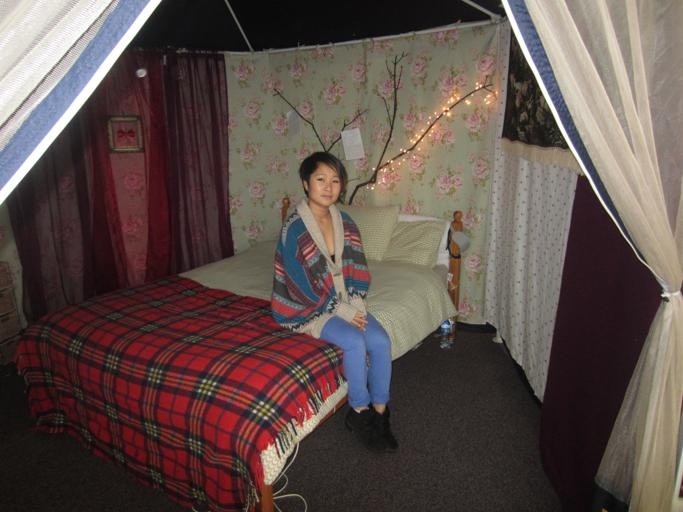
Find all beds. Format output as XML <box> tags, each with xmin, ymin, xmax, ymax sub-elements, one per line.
<box><xmin>8</xmin><ymin>197</ymin><xmax>463</xmax><ymax>512</ymax></box>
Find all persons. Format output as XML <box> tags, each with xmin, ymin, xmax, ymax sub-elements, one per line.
<box><xmin>270</xmin><ymin>151</ymin><xmax>401</xmax><ymax>453</ymax></box>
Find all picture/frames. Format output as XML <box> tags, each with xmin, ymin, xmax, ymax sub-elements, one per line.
<box><xmin>106</xmin><ymin>115</ymin><xmax>145</xmax><ymax>153</ymax></box>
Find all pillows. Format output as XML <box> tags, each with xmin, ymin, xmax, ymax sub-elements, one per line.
<box><xmin>286</xmin><ymin>204</ymin><xmax>451</xmax><ymax>269</ymax></box>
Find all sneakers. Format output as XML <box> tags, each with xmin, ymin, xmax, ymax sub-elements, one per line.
<box><xmin>345</xmin><ymin>402</ymin><xmax>400</xmax><ymax>453</ymax></box>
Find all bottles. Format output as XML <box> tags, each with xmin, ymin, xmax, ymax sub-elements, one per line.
<box><xmin>439</xmin><ymin>317</ymin><xmax>455</xmax><ymax>350</ymax></box>
